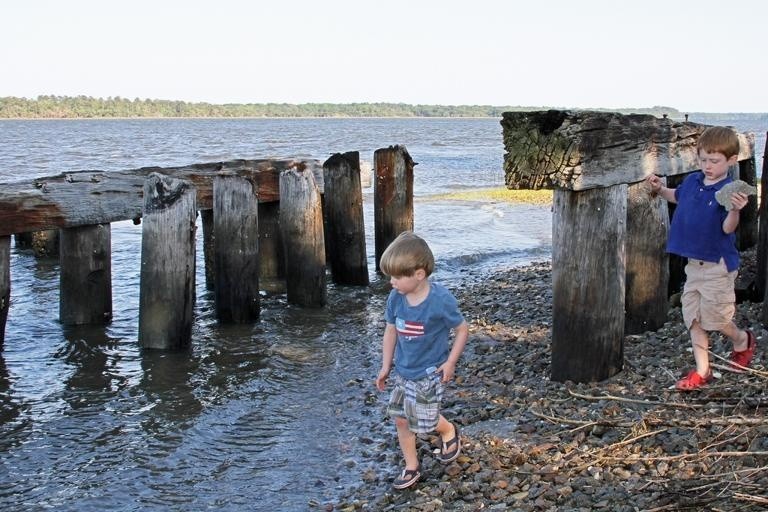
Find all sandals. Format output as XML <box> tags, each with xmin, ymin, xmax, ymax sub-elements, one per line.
<box><xmin>728</xmin><ymin>329</ymin><xmax>757</xmax><ymax>374</ymax></box>
<box><xmin>438</xmin><ymin>420</ymin><xmax>461</xmax><ymax>463</ymax></box>
<box><xmin>675</xmin><ymin>368</ymin><xmax>714</xmax><ymax>392</ymax></box>
<box><xmin>391</xmin><ymin>461</ymin><xmax>425</xmax><ymax>489</ymax></box>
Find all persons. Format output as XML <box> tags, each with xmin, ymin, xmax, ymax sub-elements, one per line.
<box><xmin>373</xmin><ymin>232</ymin><xmax>472</xmax><ymax>490</ymax></box>
<box><xmin>645</xmin><ymin>123</ymin><xmax>757</xmax><ymax>394</ymax></box>
